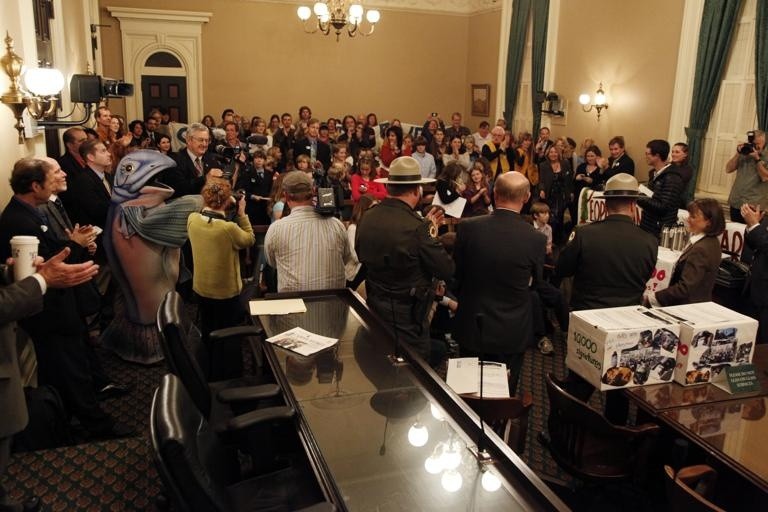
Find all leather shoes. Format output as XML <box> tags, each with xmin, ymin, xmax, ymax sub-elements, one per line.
<box><xmin>99</xmin><ymin>381</ymin><xmax>137</xmax><ymax>402</ymax></box>
<box><xmin>107</xmin><ymin>422</ymin><xmax>136</xmax><ymax>438</ymax></box>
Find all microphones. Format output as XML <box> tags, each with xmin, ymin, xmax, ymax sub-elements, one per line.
<box><xmin>477</xmin><ymin>313</ymin><xmax>485</xmax><ymax>452</ymax></box>
<box><xmin>383</xmin><ymin>254</ymin><xmax>400</xmax><ymax>362</ymax></box>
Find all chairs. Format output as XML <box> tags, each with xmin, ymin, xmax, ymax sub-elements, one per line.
<box><xmin>535</xmin><ymin>371</ymin><xmax>727</xmax><ymax>511</ymax></box>
<box><xmin>714</xmin><ymin>260</ymin><xmax>751</xmax><ymax>307</ymax></box>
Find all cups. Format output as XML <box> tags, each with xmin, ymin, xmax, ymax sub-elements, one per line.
<box><xmin>8</xmin><ymin>234</ymin><xmax>40</xmax><ymax>280</ymax></box>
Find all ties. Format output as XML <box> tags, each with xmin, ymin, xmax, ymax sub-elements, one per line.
<box><xmin>55</xmin><ymin>199</ymin><xmax>73</xmax><ymax>233</ymax></box>
<box><xmin>102</xmin><ymin>177</ymin><xmax>112</xmax><ymax>197</ymax></box>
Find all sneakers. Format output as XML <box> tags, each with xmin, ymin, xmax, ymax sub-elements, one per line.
<box><xmin>537</xmin><ymin>337</ymin><xmax>555</xmax><ymax>354</ymax></box>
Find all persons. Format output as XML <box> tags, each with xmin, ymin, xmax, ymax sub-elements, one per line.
<box><xmin>0</xmin><ymin>247</ymin><xmax>99</xmax><ymax>510</ymax></box>
<box><xmin>1</xmin><ymin>102</ymin><xmax>768</xmax><ymax>451</ymax></box>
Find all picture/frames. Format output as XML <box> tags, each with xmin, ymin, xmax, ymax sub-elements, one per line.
<box><xmin>470</xmin><ymin>83</ymin><xmax>490</xmax><ymax>117</ymax></box>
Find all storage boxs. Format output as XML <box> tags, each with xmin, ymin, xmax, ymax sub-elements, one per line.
<box><xmin>561</xmin><ymin>299</ymin><xmax>761</xmax><ymax>394</ymax></box>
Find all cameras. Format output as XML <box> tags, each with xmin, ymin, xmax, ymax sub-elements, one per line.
<box><xmin>741</xmin><ymin>131</ymin><xmax>756</xmax><ymax>154</ymax></box>
<box><xmin>214</xmin><ymin>144</ymin><xmax>234</xmax><ymax>158</ymax></box>
<box><xmin>229</xmin><ymin>188</ymin><xmax>246</xmax><ymax>207</ymax></box>
<box><xmin>432</xmin><ymin>113</ymin><xmax>437</xmax><ymax>117</ymax></box>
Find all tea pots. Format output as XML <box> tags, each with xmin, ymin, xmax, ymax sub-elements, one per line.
<box><xmin>660</xmin><ymin>221</ymin><xmax>690</xmax><ymax>250</ymax></box>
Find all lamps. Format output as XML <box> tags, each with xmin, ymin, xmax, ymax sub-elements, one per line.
<box><xmin>297</xmin><ymin>0</ymin><xmax>381</xmax><ymax>44</ymax></box>
<box><xmin>578</xmin><ymin>82</ymin><xmax>608</xmax><ymax>123</ymax></box>
<box><xmin>0</xmin><ymin>29</ymin><xmax>66</xmax><ymax>146</ymax></box>
<box><xmin>534</xmin><ymin>89</ymin><xmax>563</xmax><ymax>117</ymax></box>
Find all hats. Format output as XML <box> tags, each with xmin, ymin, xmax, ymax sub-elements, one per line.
<box><xmin>373</xmin><ymin>155</ymin><xmax>438</xmax><ymax>184</ymax></box>
<box><xmin>593</xmin><ymin>172</ymin><xmax>651</xmax><ymax>199</ymax></box>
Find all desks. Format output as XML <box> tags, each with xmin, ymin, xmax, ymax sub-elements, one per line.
<box><xmin>642</xmin><ymin>247</ymin><xmax>683</xmax><ymax>293</ymax></box>
<box><xmin>629</xmin><ymin>370</ymin><xmax>766</xmax><ymax>497</ymax></box>
<box><xmin>246</xmin><ymin>285</ymin><xmax>584</xmax><ymax>511</ymax></box>
